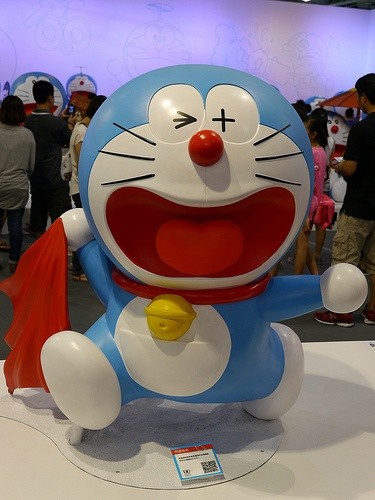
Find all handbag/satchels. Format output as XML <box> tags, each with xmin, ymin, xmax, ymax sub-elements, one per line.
<box><xmin>60</xmin><ymin>151</ymin><xmax>73</xmax><ymax>181</ymax></box>
<box><xmin>309</xmin><ymin>192</ymin><xmax>335</xmax><ymax>232</ymax></box>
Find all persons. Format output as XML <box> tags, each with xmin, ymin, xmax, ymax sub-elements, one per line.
<box><xmin>293</xmin><ymin>113</ymin><xmax>329</xmax><ymax>276</ymax></box>
<box><xmin>314</xmin><ymin>72</ymin><xmax>375</xmax><ymax>327</ymax></box>
<box><xmin>0</xmin><ymin>94</ymin><xmax>36</xmax><ymax>274</ymax></box>
<box><xmin>25</xmin><ymin>80</ymin><xmax>73</xmax><ymax>245</ymax></box>
<box><xmin>70</xmin><ymin>95</ymin><xmax>107</xmax><ymax>281</ymax></box>
<box><xmin>344</xmin><ymin>107</ymin><xmax>357</xmax><ymax>126</ymax></box>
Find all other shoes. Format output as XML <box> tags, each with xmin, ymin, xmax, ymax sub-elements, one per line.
<box><xmin>288</xmin><ymin>256</ymin><xmax>296</xmax><ymax>263</ymax></box>
<box><xmin>22</xmin><ymin>228</ymin><xmax>41</xmax><ymax>237</ymax></box>
<box><xmin>71</xmin><ymin>273</ymin><xmax>88</xmax><ymax>281</ymax></box>
<box><xmin>0</xmin><ymin>239</ymin><xmax>10</xmax><ymax>252</ymax></box>
<box><xmin>68</xmin><ymin>262</ymin><xmax>74</xmax><ymax>270</ymax></box>
<box><xmin>9</xmin><ymin>264</ymin><xmax>17</xmax><ymax>273</ymax></box>
<box><xmin>316</xmin><ymin>256</ymin><xmax>322</xmax><ymax>265</ymax></box>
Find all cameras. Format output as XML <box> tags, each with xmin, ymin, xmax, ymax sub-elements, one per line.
<box><xmin>68</xmin><ymin>105</ymin><xmax>75</xmax><ymax>115</ymax></box>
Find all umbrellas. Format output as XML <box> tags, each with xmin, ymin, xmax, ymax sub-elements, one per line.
<box><xmin>315</xmin><ymin>88</ymin><xmax>362</xmax><ymax>121</ymax></box>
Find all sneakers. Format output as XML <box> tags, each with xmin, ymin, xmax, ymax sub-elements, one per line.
<box><xmin>362</xmin><ymin>304</ymin><xmax>375</xmax><ymax>324</ymax></box>
<box><xmin>312</xmin><ymin>310</ymin><xmax>354</xmax><ymax>327</ymax></box>
